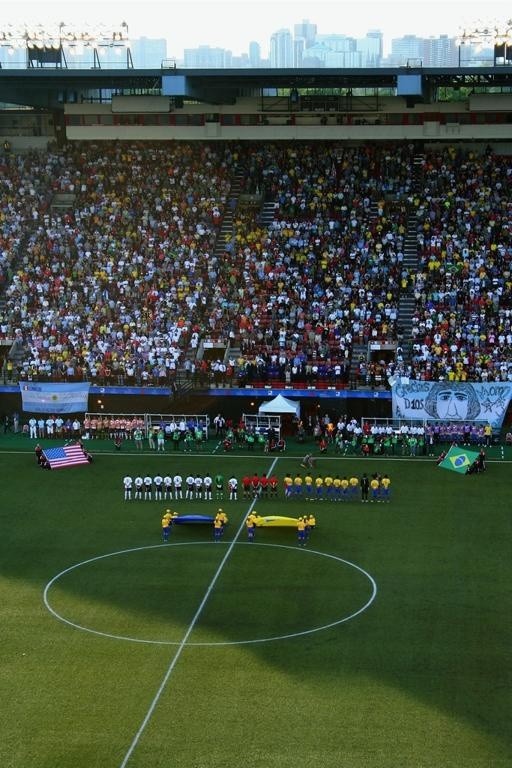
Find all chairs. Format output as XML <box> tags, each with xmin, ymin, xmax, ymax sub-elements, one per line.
<box><xmin>208</xmin><ymin>271</ymin><xmax>352</xmax><ymax>372</ymax></box>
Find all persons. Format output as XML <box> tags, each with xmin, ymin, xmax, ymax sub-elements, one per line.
<box><xmin>162</xmin><ymin>515</ymin><xmax>170</xmax><ymax>543</ymax></box>
<box><xmin>0</xmin><ymin>139</ymin><xmax>512</xmax><ymax>457</ymax></box>
<box><xmin>36</xmin><ymin>454</ymin><xmax>51</xmax><ymax>472</ymax></box>
<box><xmin>249</xmin><ymin>511</ymin><xmax>257</xmax><ymax>538</ymax></box>
<box><xmin>308</xmin><ymin>514</ymin><xmax>315</xmax><ymax>529</ymax></box>
<box><xmin>299</xmin><ymin>453</ymin><xmax>313</xmax><ymax>471</ymax></box>
<box><xmin>435</xmin><ymin>455</ymin><xmax>444</xmax><ymax>466</ymax></box>
<box><xmin>165</xmin><ymin>509</ymin><xmax>179</xmax><ymax>529</ymax></box>
<box><xmin>467</xmin><ymin>451</ymin><xmax>487</xmax><ymax>478</ymax></box>
<box><xmin>216</xmin><ymin>508</ymin><xmax>228</xmax><ymax>527</ymax></box>
<box><xmin>297</xmin><ymin>517</ymin><xmax>306</xmax><ymax>547</ymax></box>
<box><xmin>246</xmin><ymin>516</ymin><xmax>255</xmax><ymax>544</ymax></box>
<box><xmin>85</xmin><ymin>452</ymin><xmax>93</xmax><ymax>464</ymax></box>
<box><xmin>213</xmin><ymin>516</ymin><xmax>222</xmax><ymax>543</ymax></box>
<box><xmin>122</xmin><ymin>471</ymin><xmax>391</xmax><ymax>505</ymax></box>
<box><xmin>303</xmin><ymin>515</ymin><xmax>310</xmax><ymax>541</ymax></box>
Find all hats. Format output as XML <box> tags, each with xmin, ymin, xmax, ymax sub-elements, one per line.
<box><xmin>215</xmin><ymin>508</ymin><xmax>223</xmax><ymax>520</ymax></box>
<box><xmin>247</xmin><ymin>510</ymin><xmax>257</xmax><ymax>519</ymax></box>
<box><xmin>299</xmin><ymin>514</ymin><xmax>314</xmax><ymax>521</ymax></box>
<box><xmin>163</xmin><ymin>508</ymin><xmax>171</xmax><ymax>519</ymax></box>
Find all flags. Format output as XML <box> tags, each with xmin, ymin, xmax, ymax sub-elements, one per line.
<box><xmin>172</xmin><ymin>512</ymin><xmax>216</xmax><ymax>525</ymax></box>
<box><xmin>254</xmin><ymin>515</ymin><xmax>302</xmax><ymax>528</ymax></box>
<box><xmin>436</xmin><ymin>445</ymin><xmax>481</xmax><ymax>475</ymax></box>
<box><xmin>41</xmin><ymin>443</ymin><xmax>90</xmax><ymax>468</ymax></box>
